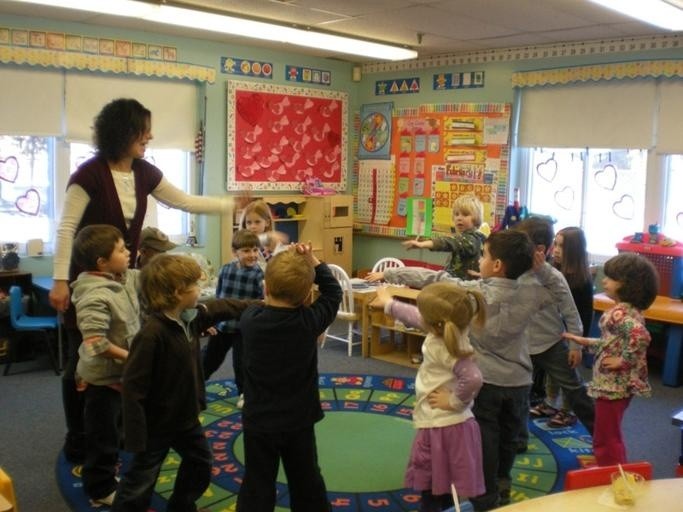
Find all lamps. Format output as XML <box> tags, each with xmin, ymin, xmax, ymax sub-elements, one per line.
<box><xmin>143</xmin><ymin>5</ymin><xmax>419</xmax><ymax>63</ymax></box>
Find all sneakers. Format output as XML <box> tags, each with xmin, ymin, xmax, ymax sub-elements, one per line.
<box><xmin>530</xmin><ymin>402</ymin><xmax>558</xmax><ymax>419</ymax></box>
<box><xmin>547</xmin><ymin>410</ymin><xmax>577</xmax><ymax>428</ymax></box>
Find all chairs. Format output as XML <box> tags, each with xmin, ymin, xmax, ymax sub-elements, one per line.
<box><xmin>369</xmin><ymin>257</ymin><xmax>409</xmax><ymax>345</ymax></box>
<box><xmin>2</xmin><ymin>286</ymin><xmax>62</xmax><ymax>374</ymax></box>
<box><xmin>316</xmin><ymin>265</ymin><xmax>370</xmax><ymax>357</ymax></box>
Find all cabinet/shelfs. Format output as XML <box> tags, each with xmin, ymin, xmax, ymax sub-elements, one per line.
<box><xmin>220</xmin><ymin>194</ymin><xmax>356</xmax><ymax>284</ymax></box>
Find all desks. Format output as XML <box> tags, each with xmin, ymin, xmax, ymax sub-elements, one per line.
<box><xmin>31</xmin><ymin>275</ymin><xmax>68</xmax><ymax>364</ymax></box>
<box><xmin>335</xmin><ymin>277</ymin><xmax>404</xmax><ymax>360</ymax></box>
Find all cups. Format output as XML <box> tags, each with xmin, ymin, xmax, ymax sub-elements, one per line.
<box><xmin>407</xmin><ymin>341</ymin><xmax>422</xmax><ymax>364</ymax></box>
<box><xmin>611</xmin><ymin>471</ymin><xmax>645</xmax><ymax>506</ymax></box>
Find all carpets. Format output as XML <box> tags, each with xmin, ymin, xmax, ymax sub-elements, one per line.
<box><xmin>57</xmin><ymin>373</ymin><xmax>597</xmax><ymax>512</ymax></box>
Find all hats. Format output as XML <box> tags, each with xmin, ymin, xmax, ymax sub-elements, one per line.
<box><xmin>137</xmin><ymin>223</ymin><xmax>181</xmax><ymax>253</ymax></box>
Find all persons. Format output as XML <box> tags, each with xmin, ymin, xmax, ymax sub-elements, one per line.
<box><xmin>231</xmin><ymin>240</ymin><xmax>344</xmax><ymax>512</ymax></box>
<box><xmin>134</xmin><ymin>225</ymin><xmax>177</xmax><ymax>269</ymax></box>
<box><xmin>47</xmin><ymin>100</ymin><xmax>252</xmax><ymax>464</ymax></box>
<box><xmin>561</xmin><ymin>252</ymin><xmax>659</xmax><ymax>467</ymax></box>
<box><xmin>70</xmin><ymin>225</ymin><xmax>153</xmax><ymax>506</ymax></box>
<box><xmin>365</xmin><ymin>230</ymin><xmax>556</xmax><ymax>511</ymax></box>
<box><xmin>375</xmin><ymin>285</ymin><xmax>486</xmax><ymax>512</ymax></box>
<box><xmin>201</xmin><ymin>229</ymin><xmax>265</xmax><ymax>409</ymax></box>
<box><xmin>400</xmin><ymin>194</ymin><xmax>491</xmax><ymax>281</ymax></box>
<box><xmin>110</xmin><ymin>253</ymin><xmax>265</xmax><ymax>512</ymax></box>
<box><xmin>529</xmin><ymin>227</ymin><xmax>593</xmax><ymax>428</ymax></box>
<box><xmin>512</xmin><ymin>218</ymin><xmax>596</xmax><ymax>436</ymax></box>
<box><xmin>239</xmin><ymin>200</ymin><xmax>283</xmax><ymax>271</ymax></box>
